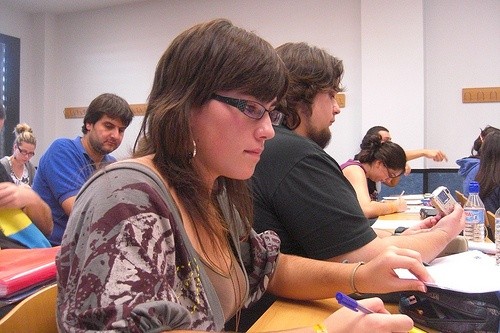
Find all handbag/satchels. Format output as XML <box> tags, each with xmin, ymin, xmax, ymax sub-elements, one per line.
<box><xmin>348</xmin><ymin>285</ymin><xmax>500</xmax><ymax>333</ymax></box>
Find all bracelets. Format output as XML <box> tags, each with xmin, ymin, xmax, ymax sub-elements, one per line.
<box><xmin>313</xmin><ymin>323</ymin><xmax>328</xmax><ymax>333</ymax></box>
<box><xmin>350</xmin><ymin>261</ymin><xmax>366</xmax><ymax>295</ymax></box>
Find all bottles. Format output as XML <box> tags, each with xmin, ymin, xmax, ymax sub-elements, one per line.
<box><xmin>463</xmin><ymin>182</ymin><xmax>485</xmax><ymax>251</ymax></box>
<box><xmin>495</xmin><ymin>208</ymin><xmax>500</xmax><ymax>266</ymax></box>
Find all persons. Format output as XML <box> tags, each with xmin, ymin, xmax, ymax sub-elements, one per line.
<box><xmin>0</xmin><ymin>123</ymin><xmax>37</xmax><ymax>188</ymax></box>
<box><xmin>476</xmin><ymin>134</ymin><xmax>500</xmax><ymax>215</ymax></box>
<box><xmin>0</xmin><ymin>181</ymin><xmax>54</xmax><ymax>249</ymax></box>
<box><xmin>56</xmin><ymin>17</ymin><xmax>429</xmax><ymax>333</ymax></box>
<box><xmin>455</xmin><ymin>126</ymin><xmax>500</xmax><ymax>200</ymax></box>
<box><xmin>30</xmin><ymin>93</ymin><xmax>133</xmax><ymax>247</ymax></box>
<box><xmin>231</xmin><ymin>42</ymin><xmax>466</xmax><ymax>266</ymax></box>
<box><xmin>360</xmin><ymin>126</ymin><xmax>448</xmax><ymax>201</ymax></box>
<box><xmin>0</xmin><ymin>96</ymin><xmax>7</xmax><ymax>129</ymax></box>
<box><xmin>339</xmin><ymin>133</ymin><xmax>408</xmax><ymax>219</ymax></box>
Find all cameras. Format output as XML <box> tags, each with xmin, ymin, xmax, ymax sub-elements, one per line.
<box><xmin>431</xmin><ymin>185</ymin><xmax>459</xmax><ymax>216</ymax></box>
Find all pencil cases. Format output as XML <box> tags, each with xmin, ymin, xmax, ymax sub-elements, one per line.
<box><xmin>399</xmin><ymin>286</ymin><xmax>500</xmax><ymax>333</ymax></box>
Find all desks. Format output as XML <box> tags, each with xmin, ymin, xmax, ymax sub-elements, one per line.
<box><xmin>245</xmin><ymin>199</ymin><xmax>494</xmax><ymax>333</ymax></box>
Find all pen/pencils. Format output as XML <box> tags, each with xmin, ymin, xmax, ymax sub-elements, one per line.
<box><xmin>337</xmin><ymin>292</ymin><xmax>374</xmax><ymax>314</ymax></box>
<box><xmin>403</xmin><ymin>295</ymin><xmax>465</xmax><ymax>320</ymax></box>
<box><xmin>398</xmin><ymin>190</ymin><xmax>405</xmax><ymax>199</ymax></box>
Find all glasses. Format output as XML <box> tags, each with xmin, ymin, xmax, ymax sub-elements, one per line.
<box><xmin>383</xmin><ymin>163</ymin><xmax>396</xmax><ymax>179</ymax></box>
<box><xmin>211</xmin><ymin>93</ymin><xmax>284</xmax><ymax>126</ymax></box>
<box><xmin>17</xmin><ymin>146</ymin><xmax>34</xmax><ymax>157</ymax></box>
<box><xmin>320</xmin><ymin>87</ymin><xmax>346</xmax><ymax>108</ymax></box>
